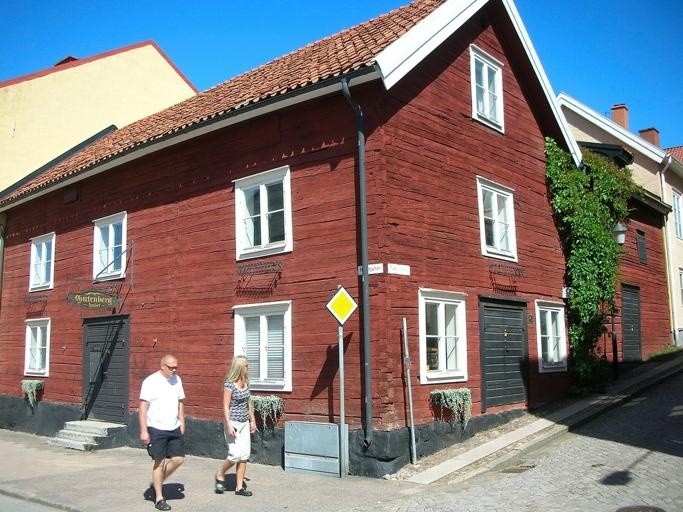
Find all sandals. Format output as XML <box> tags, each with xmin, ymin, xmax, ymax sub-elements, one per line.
<box><xmin>145</xmin><ymin>487</ymin><xmax>171</xmax><ymax>512</ymax></box>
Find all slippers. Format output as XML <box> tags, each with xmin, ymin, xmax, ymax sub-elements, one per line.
<box><xmin>214</xmin><ymin>473</ymin><xmax>226</xmax><ymax>494</ymax></box>
<box><xmin>233</xmin><ymin>488</ymin><xmax>253</xmax><ymax>497</ymax></box>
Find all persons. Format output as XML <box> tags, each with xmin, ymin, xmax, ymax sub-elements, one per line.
<box><xmin>137</xmin><ymin>354</ymin><xmax>186</xmax><ymax>511</ymax></box>
<box><xmin>214</xmin><ymin>355</ymin><xmax>258</xmax><ymax>496</ymax></box>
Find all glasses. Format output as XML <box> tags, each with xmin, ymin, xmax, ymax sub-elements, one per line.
<box><xmin>161</xmin><ymin>363</ymin><xmax>178</xmax><ymax>372</ymax></box>
<box><xmin>243</xmin><ymin>364</ymin><xmax>248</xmax><ymax>367</ymax></box>
